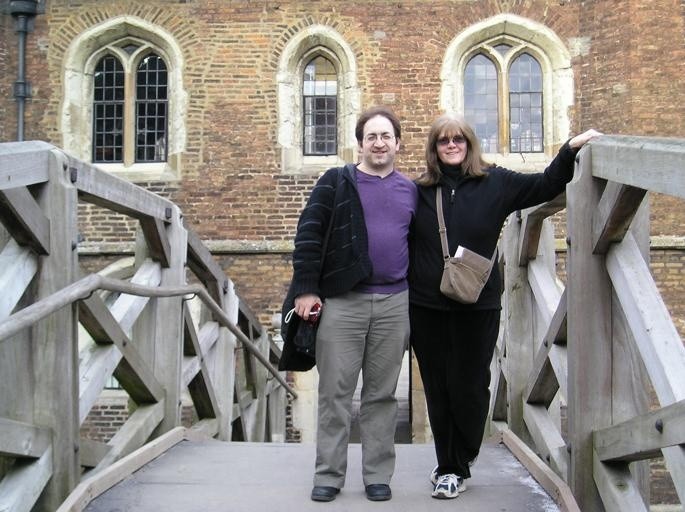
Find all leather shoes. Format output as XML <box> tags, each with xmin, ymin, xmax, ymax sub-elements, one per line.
<box><xmin>311</xmin><ymin>484</ymin><xmax>340</xmax><ymax>502</ymax></box>
<box><xmin>365</xmin><ymin>481</ymin><xmax>392</xmax><ymax>501</ymax></box>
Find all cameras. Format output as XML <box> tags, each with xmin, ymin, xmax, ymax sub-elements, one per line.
<box><xmin>308</xmin><ymin>303</ymin><xmax>321</xmax><ymax>322</ymax></box>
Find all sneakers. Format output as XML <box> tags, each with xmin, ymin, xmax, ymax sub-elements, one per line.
<box><xmin>430</xmin><ymin>463</ymin><xmax>468</xmax><ymax>499</ymax></box>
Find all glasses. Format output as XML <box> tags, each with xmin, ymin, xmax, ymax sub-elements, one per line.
<box><xmin>435</xmin><ymin>135</ymin><xmax>468</xmax><ymax>146</ymax></box>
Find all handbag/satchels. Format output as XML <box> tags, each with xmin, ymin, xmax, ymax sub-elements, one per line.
<box><xmin>438</xmin><ymin>248</ymin><xmax>496</xmax><ymax>307</ymax></box>
<box><xmin>276</xmin><ymin>279</ymin><xmax>327</xmax><ymax>373</ymax></box>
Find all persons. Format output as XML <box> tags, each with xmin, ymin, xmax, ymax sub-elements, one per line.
<box><xmin>292</xmin><ymin>104</ymin><xmax>418</xmax><ymax>501</ymax></box>
<box><xmin>407</xmin><ymin>112</ymin><xmax>606</xmax><ymax>500</ymax></box>
